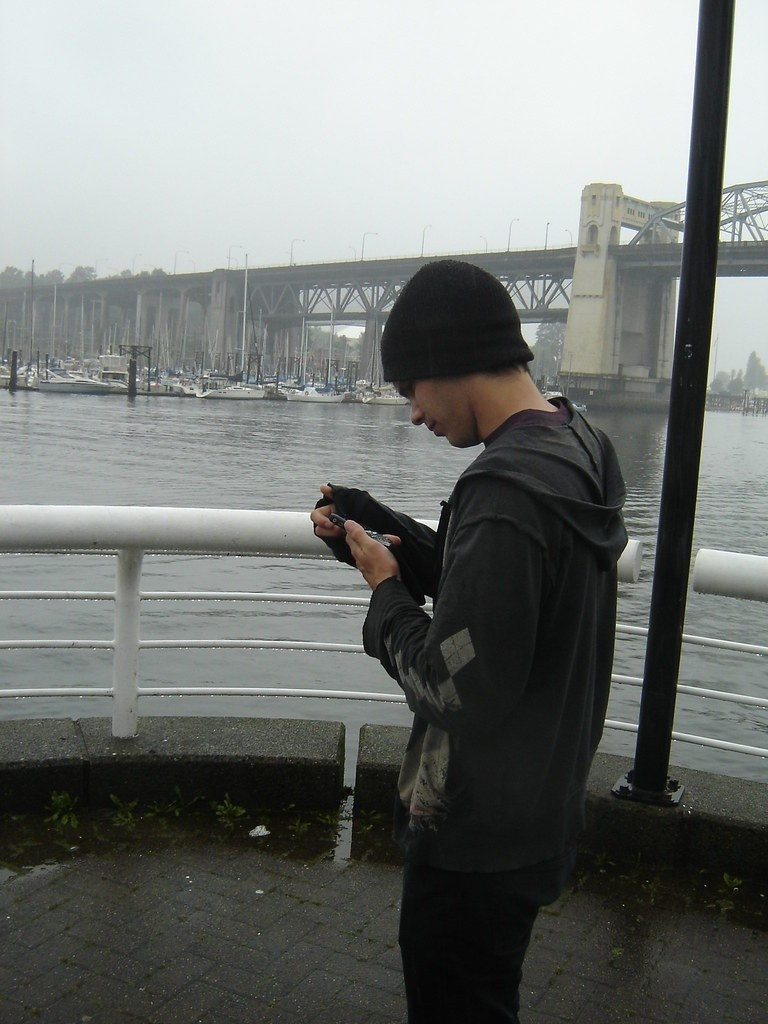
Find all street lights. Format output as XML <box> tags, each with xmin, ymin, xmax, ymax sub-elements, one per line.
<box><xmin>546</xmin><ymin>222</ymin><xmax>551</xmax><ymax>249</ymax></box>
<box><xmin>481</xmin><ymin>236</ymin><xmax>487</xmax><ymax>253</ymax></box>
<box><xmin>566</xmin><ymin>229</ymin><xmax>572</xmax><ymax>247</ymax></box>
<box><xmin>421</xmin><ymin>225</ymin><xmax>433</xmax><ymax>256</ymax></box>
<box><xmin>362</xmin><ymin>233</ymin><xmax>378</xmax><ymax>260</ymax></box>
<box><xmin>508</xmin><ymin>218</ymin><xmax>520</xmax><ymax>251</ymax></box>
<box><xmin>290</xmin><ymin>239</ymin><xmax>306</xmax><ymax>265</ymax></box>
<box><xmin>228</xmin><ymin>245</ymin><xmax>243</xmax><ymax>270</ymax></box>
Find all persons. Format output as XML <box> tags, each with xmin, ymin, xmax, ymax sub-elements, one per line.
<box><xmin>310</xmin><ymin>261</ymin><xmax>630</xmax><ymax>1024</ymax></box>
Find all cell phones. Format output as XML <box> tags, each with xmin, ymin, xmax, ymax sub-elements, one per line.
<box><xmin>328</xmin><ymin>512</ymin><xmax>395</xmax><ymax>549</ymax></box>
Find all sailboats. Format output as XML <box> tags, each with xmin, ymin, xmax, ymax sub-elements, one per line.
<box><xmin>0</xmin><ymin>258</ymin><xmax>195</xmax><ymax>396</ymax></box>
<box><xmin>530</xmin><ymin>350</ymin><xmax>588</xmax><ymax>411</ymax></box>
<box><xmin>197</xmin><ymin>253</ymin><xmax>410</xmax><ymax>405</ymax></box>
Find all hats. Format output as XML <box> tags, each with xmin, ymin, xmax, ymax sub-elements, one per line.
<box><xmin>380</xmin><ymin>260</ymin><xmax>534</xmax><ymax>383</ymax></box>
